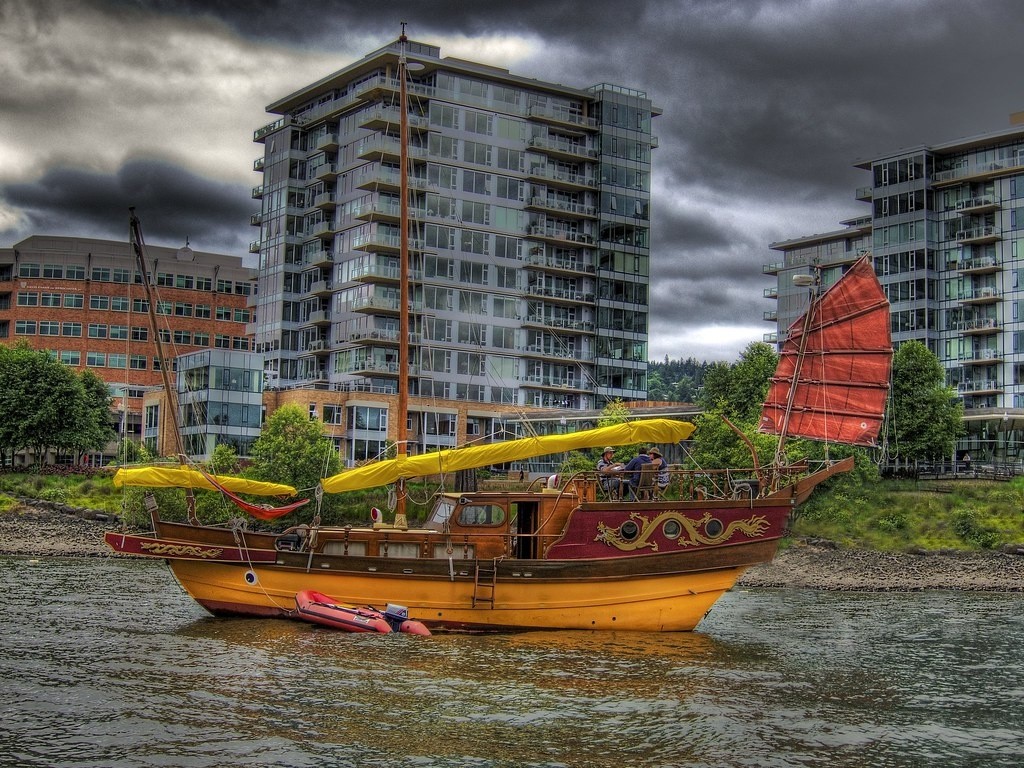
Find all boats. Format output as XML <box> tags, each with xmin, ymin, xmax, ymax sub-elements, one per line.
<box><xmin>292</xmin><ymin>591</ymin><xmax>433</xmax><ymax>636</ymax></box>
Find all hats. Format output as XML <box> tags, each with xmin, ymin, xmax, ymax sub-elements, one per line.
<box><xmin>601</xmin><ymin>446</ymin><xmax>617</xmax><ymax>457</ymax></box>
<box><xmin>647</xmin><ymin>447</ymin><xmax>663</xmax><ymax>456</ymax></box>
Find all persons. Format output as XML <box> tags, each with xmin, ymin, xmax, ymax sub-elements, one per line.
<box><xmin>624</xmin><ymin>446</ymin><xmax>652</xmax><ymax>502</ymax></box>
<box><xmin>83</xmin><ymin>453</ymin><xmax>89</xmax><ymax>468</ymax></box>
<box><xmin>647</xmin><ymin>447</ymin><xmax>670</xmax><ymax>501</ymax></box>
<box><xmin>597</xmin><ymin>446</ymin><xmax>629</xmax><ymax>500</ymax></box>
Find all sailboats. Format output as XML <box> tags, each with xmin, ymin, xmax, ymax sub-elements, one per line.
<box><xmin>103</xmin><ymin>17</ymin><xmax>896</xmax><ymax>633</ymax></box>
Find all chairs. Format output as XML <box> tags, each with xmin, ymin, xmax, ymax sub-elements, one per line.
<box><xmin>595</xmin><ymin>475</ymin><xmax>621</xmax><ymax>502</ymax></box>
<box><xmin>633</xmin><ymin>463</ymin><xmax>660</xmax><ymax>502</ymax></box>
<box><xmin>658</xmin><ymin>466</ymin><xmax>681</xmax><ymax>500</ymax></box>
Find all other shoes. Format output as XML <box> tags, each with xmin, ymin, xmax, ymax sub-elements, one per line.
<box><xmin>613</xmin><ymin>494</ymin><xmax>619</xmax><ymax>500</ymax></box>
